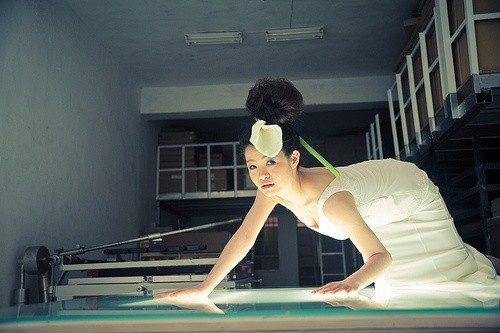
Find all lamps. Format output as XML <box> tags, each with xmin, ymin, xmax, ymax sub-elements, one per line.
<box><xmin>265</xmin><ymin>25</ymin><xmax>324</xmax><ymax>42</ymax></box>
<box><xmin>184</xmin><ymin>32</ymin><xmax>242</xmax><ymax>46</ymax></box>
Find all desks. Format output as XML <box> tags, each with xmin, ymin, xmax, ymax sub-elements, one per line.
<box><xmin>0</xmin><ymin>286</ymin><xmax>500</xmax><ymax>333</ymax></box>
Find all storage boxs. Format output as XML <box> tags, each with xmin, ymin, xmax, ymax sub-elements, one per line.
<box><xmin>159</xmin><ymin>129</ymin><xmax>227</xmax><ymax>194</ymax></box>
<box><xmin>393</xmin><ymin>0</ymin><xmax>500</xmax><ymax>130</ymax></box>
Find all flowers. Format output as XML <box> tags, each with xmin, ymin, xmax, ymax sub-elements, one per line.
<box><xmin>249</xmin><ymin>116</ymin><xmax>340</xmax><ymax>177</ymax></box>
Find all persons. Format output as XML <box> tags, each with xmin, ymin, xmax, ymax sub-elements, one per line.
<box><xmin>154</xmin><ymin>78</ymin><xmax>500</xmax><ymax>312</ymax></box>
